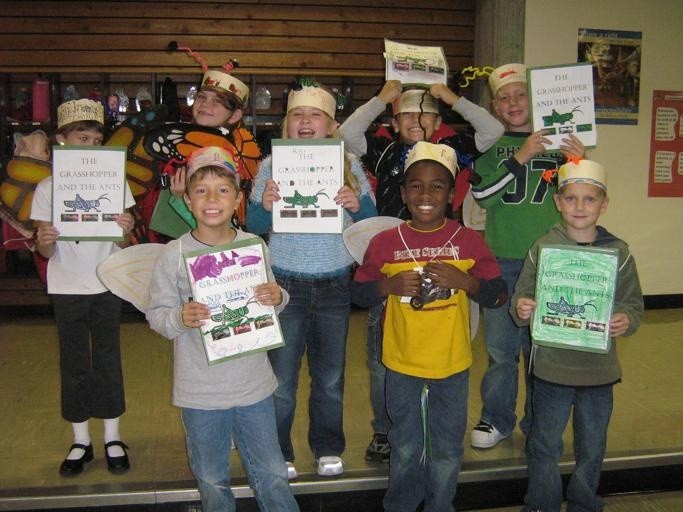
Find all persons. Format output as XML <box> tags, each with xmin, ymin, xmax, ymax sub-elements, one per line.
<box><xmin>469</xmin><ymin>63</ymin><xmax>585</xmax><ymax>451</ymax></box>
<box><xmin>30</xmin><ymin>98</ymin><xmax>135</xmax><ymax>477</ymax></box>
<box><xmin>246</xmin><ymin>80</ymin><xmax>378</xmax><ymax>482</ymax></box>
<box><xmin>337</xmin><ymin>79</ymin><xmax>505</xmax><ymax>463</ymax></box>
<box><xmin>350</xmin><ymin>142</ymin><xmax>509</xmax><ymax>512</ymax></box>
<box><xmin>146</xmin><ymin>145</ymin><xmax>302</xmax><ymax>511</ymax></box>
<box><xmin>509</xmin><ymin>158</ymin><xmax>643</xmax><ymax>512</ymax></box>
<box><xmin>148</xmin><ymin>68</ymin><xmax>263</xmax><ymax>245</ymax></box>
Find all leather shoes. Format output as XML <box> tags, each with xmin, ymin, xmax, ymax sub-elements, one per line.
<box><xmin>58</xmin><ymin>440</ymin><xmax>94</xmax><ymax>478</ymax></box>
<box><xmin>104</xmin><ymin>438</ymin><xmax>130</xmax><ymax>475</ymax></box>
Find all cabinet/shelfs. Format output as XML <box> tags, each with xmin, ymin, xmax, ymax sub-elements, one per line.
<box><xmin>0</xmin><ymin>70</ymin><xmax>475</xmax><ymax>305</ymax></box>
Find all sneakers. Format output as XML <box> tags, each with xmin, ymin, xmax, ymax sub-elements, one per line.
<box><xmin>470</xmin><ymin>419</ymin><xmax>508</xmax><ymax>450</ymax></box>
<box><xmin>314</xmin><ymin>454</ymin><xmax>345</xmax><ymax>476</ymax></box>
<box><xmin>285</xmin><ymin>460</ymin><xmax>299</xmax><ymax>480</ymax></box>
<box><xmin>364</xmin><ymin>432</ymin><xmax>392</xmax><ymax>463</ymax></box>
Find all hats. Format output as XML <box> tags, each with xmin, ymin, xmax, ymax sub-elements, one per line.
<box><xmin>286</xmin><ymin>73</ymin><xmax>337</xmax><ymax>120</ymax></box>
<box><xmin>185</xmin><ymin>146</ymin><xmax>240</xmax><ymax>192</ymax></box>
<box><xmin>403</xmin><ymin>140</ymin><xmax>458</xmax><ymax>182</ymax></box>
<box><xmin>557</xmin><ymin>154</ymin><xmax>608</xmax><ymax>194</ymax></box>
<box><xmin>56</xmin><ymin>98</ymin><xmax>105</xmax><ymax>130</ymax></box>
<box><xmin>199</xmin><ymin>70</ymin><xmax>250</xmax><ymax>108</ymax></box>
<box><xmin>391</xmin><ymin>89</ymin><xmax>440</xmax><ymax>118</ymax></box>
<box><xmin>487</xmin><ymin>63</ymin><xmax>529</xmax><ymax>98</ymax></box>
